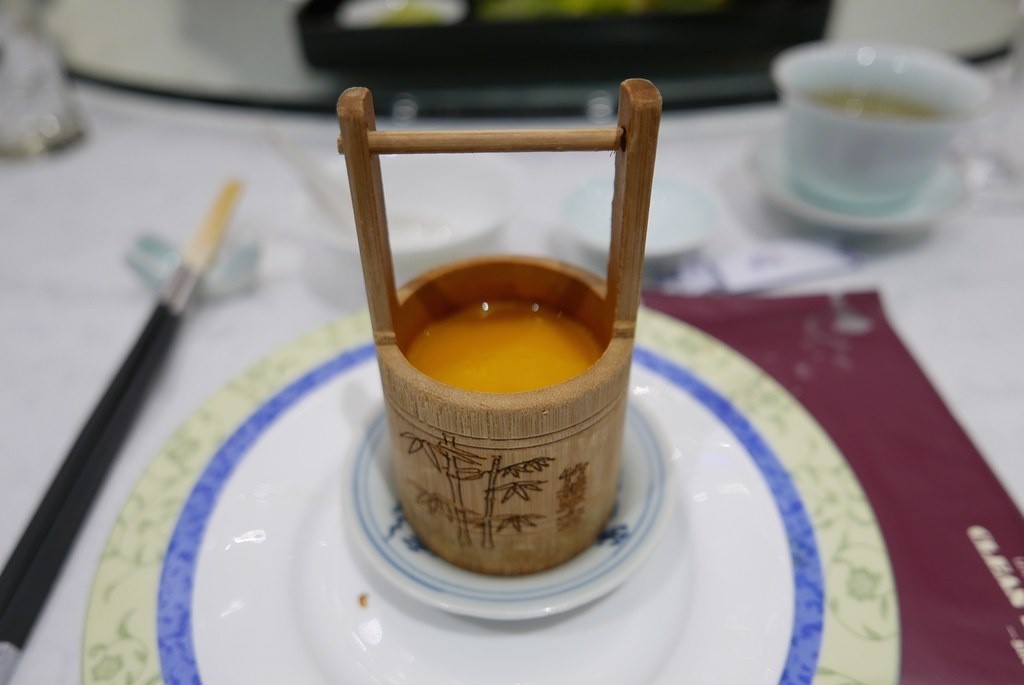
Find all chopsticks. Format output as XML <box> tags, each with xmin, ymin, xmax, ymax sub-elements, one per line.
<box><xmin>0</xmin><ymin>178</ymin><xmax>244</xmax><ymax>685</ymax></box>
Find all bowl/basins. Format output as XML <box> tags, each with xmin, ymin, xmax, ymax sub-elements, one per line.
<box><xmin>561</xmin><ymin>175</ymin><xmax>716</xmax><ymax>275</ymax></box>
<box><xmin>299</xmin><ymin>155</ymin><xmax>525</xmax><ymax>272</ymax></box>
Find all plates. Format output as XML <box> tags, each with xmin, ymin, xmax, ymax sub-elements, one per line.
<box><xmin>345</xmin><ymin>390</ymin><xmax>676</xmax><ymax>621</ymax></box>
<box><xmin>757</xmin><ymin>139</ymin><xmax>979</xmax><ymax>230</ymax></box>
<box><xmin>76</xmin><ymin>303</ymin><xmax>901</xmax><ymax>685</ymax></box>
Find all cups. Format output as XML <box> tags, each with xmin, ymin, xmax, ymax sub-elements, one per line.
<box><xmin>774</xmin><ymin>39</ymin><xmax>988</xmax><ymax>211</ymax></box>
